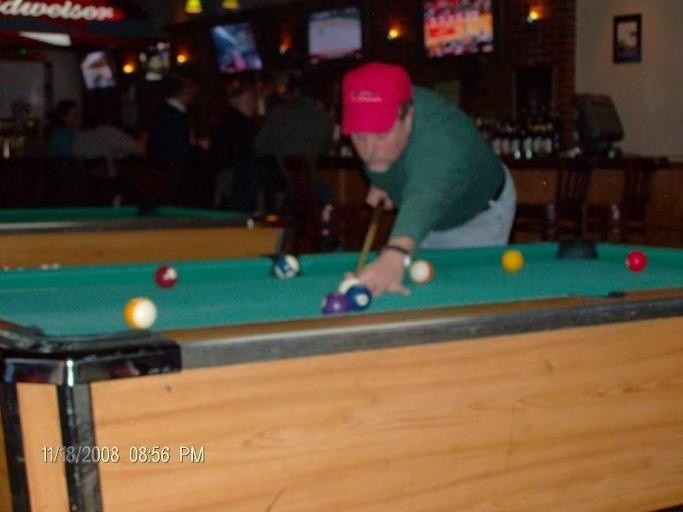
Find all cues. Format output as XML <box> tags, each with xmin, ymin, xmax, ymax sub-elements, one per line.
<box><xmin>354</xmin><ymin>199</ymin><xmax>384</xmax><ymax>276</ymax></box>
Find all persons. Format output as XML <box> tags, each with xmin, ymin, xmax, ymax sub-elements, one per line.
<box><xmin>213</xmin><ymin>85</ymin><xmax>264</xmax><ymax>170</ymax></box>
<box><xmin>39</xmin><ymin>100</ymin><xmax>138</xmax><ymax>195</ymax></box>
<box><xmin>144</xmin><ymin>74</ymin><xmax>218</xmax><ymax>196</ymax></box>
<box><xmin>339</xmin><ymin>60</ymin><xmax>517</xmax><ymax>300</ymax></box>
<box><xmin>246</xmin><ymin>72</ymin><xmax>338</xmax><ymax>208</ymax></box>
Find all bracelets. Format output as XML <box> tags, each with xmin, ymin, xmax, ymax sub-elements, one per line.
<box><xmin>375</xmin><ymin>242</ymin><xmax>413</xmax><ymax>269</ymax></box>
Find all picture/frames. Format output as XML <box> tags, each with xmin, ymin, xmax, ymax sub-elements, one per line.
<box><xmin>612</xmin><ymin>12</ymin><xmax>642</xmax><ymax>64</ymax></box>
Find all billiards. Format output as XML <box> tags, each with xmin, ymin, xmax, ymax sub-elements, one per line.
<box><xmin>624</xmin><ymin>250</ymin><xmax>647</xmax><ymax>271</ymax></box>
<box><xmin>124</xmin><ymin>297</ymin><xmax>156</xmax><ymax>330</ymax></box>
<box><xmin>155</xmin><ymin>266</ymin><xmax>176</xmax><ymax>288</ymax></box>
<box><xmin>408</xmin><ymin>260</ymin><xmax>432</xmax><ymax>284</ymax></box>
<box><xmin>273</xmin><ymin>254</ymin><xmax>299</xmax><ymax>278</ymax></box>
<box><xmin>500</xmin><ymin>248</ymin><xmax>524</xmax><ymax>272</ymax></box>
<box><xmin>320</xmin><ymin>278</ymin><xmax>372</xmax><ymax>315</ymax></box>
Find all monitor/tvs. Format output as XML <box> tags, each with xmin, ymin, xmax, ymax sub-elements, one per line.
<box><xmin>136</xmin><ymin>40</ymin><xmax>176</xmax><ymax>82</ymax></box>
<box><xmin>572</xmin><ymin>93</ymin><xmax>622</xmax><ymax>152</ymax></box>
<box><xmin>302</xmin><ymin>4</ymin><xmax>374</xmax><ymax>62</ymax></box>
<box><xmin>418</xmin><ymin>0</ymin><xmax>503</xmax><ymax>61</ymax></box>
<box><xmin>78</xmin><ymin>48</ymin><xmax>120</xmax><ymax>91</ymax></box>
<box><xmin>200</xmin><ymin>16</ymin><xmax>267</xmax><ymax>78</ymax></box>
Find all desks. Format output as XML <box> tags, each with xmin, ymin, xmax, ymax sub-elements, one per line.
<box><xmin>0</xmin><ymin>201</ymin><xmax>683</xmax><ymax>510</ymax></box>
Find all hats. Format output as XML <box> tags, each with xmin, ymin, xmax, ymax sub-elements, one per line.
<box><xmin>339</xmin><ymin>60</ymin><xmax>415</xmax><ymax>136</ymax></box>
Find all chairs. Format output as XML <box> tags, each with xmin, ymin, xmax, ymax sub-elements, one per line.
<box><xmin>506</xmin><ymin>156</ymin><xmax>654</xmax><ymax>244</ymax></box>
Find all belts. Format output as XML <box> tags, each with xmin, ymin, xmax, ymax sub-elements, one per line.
<box><xmin>480</xmin><ymin>165</ymin><xmax>508</xmax><ymax>214</ymax></box>
